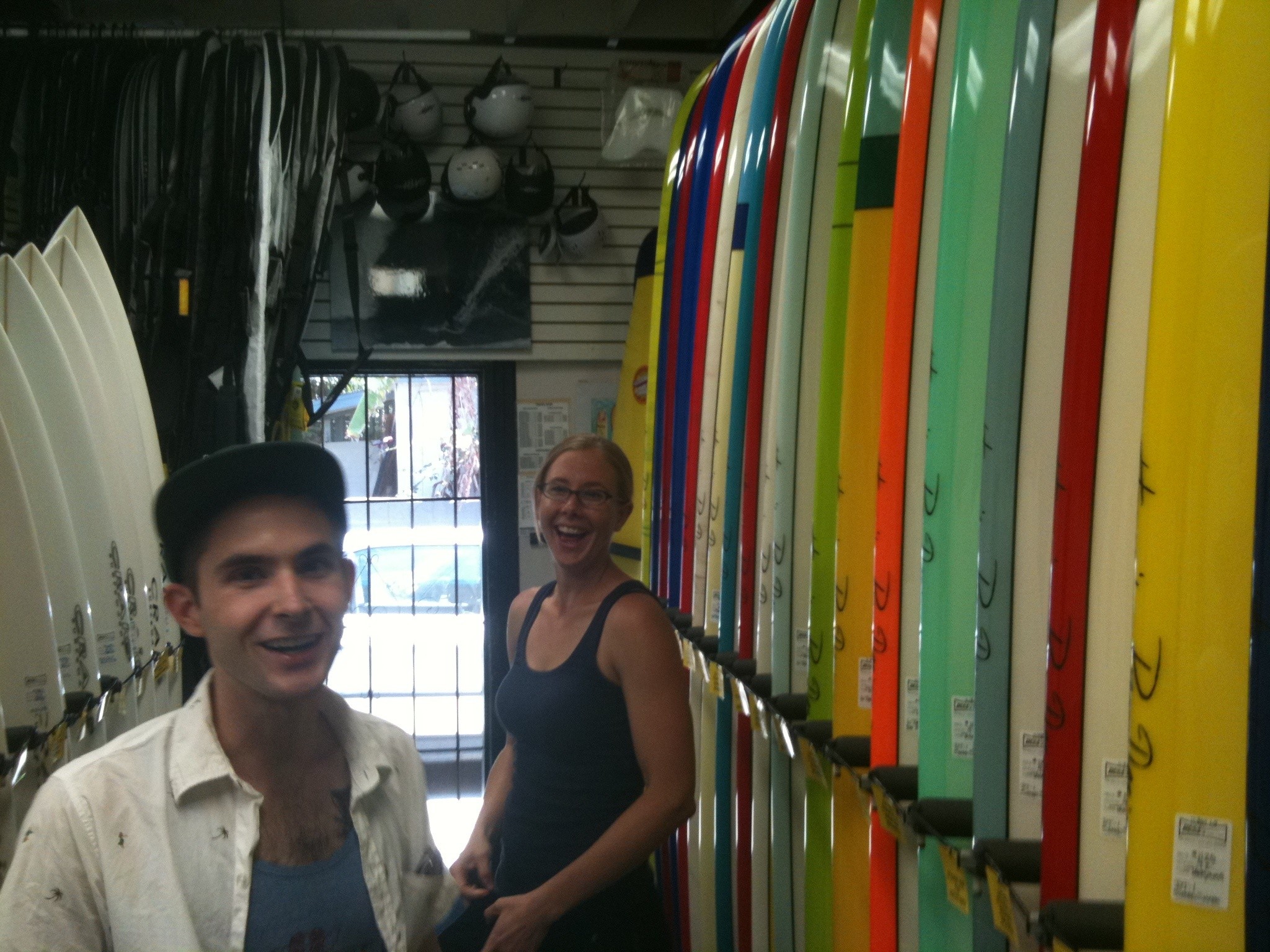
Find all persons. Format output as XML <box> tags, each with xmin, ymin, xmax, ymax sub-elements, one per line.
<box><xmin>449</xmin><ymin>433</ymin><xmax>699</xmax><ymax>952</ymax></box>
<box><xmin>0</xmin><ymin>440</ymin><xmax>460</xmax><ymax>952</ymax></box>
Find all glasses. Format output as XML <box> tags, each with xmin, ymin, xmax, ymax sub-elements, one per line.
<box><xmin>535</xmin><ymin>479</ymin><xmax>625</xmax><ymax>511</ymax></box>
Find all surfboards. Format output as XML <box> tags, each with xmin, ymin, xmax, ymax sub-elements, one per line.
<box><xmin>42</xmin><ymin>234</ymin><xmax>171</xmax><ymax>717</ymax></box>
<box><xmin>0</xmin><ymin>322</ymin><xmax>110</xmax><ymax>762</ymax></box>
<box><xmin>0</xmin><ymin>414</ymin><xmax>75</xmax><ymax>834</ymax></box>
<box><xmin>0</xmin><ymin>251</ymin><xmax>138</xmax><ymax>745</ymax></box>
<box><xmin>0</xmin><ymin>704</ymin><xmax>18</xmax><ymax>895</ymax></box>
<box><xmin>11</xmin><ymin>241</ymin><xmax>157</xmax><ymax>727</ymax></box>
<box><xmin>41</xmin><ymin>204</ymin><xmax>187</xmax><ymax>713</ymax></box>
<box><xmin>610</xmin><ymin>0</ymin><xmax>1270</xmax><ymax>952</ymax></box>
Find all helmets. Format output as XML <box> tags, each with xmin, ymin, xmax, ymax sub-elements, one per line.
<box><xmin>439</xmin><ymin>143</ymin><xmax>503</xmax><ymax>205</ymax></box>
<box><xmin>555</xmin><ymin>209</ymin><xmax>609</xmax><ymax>259</ymax></box>
<box><xmin>374</xmin><ymin>146</ymin><xmax>431</xmax><ymax>203</ymax></box>
<box><xmin>334</xmin><ymin>163</ymin><xmax>368</xmax><ymax>208</ymax></box>
<box><xmin>507</xmin><ymin>163</ymin><xmax>554</xmax><ymax>215</ymax></box>
<box><xmin>343</xmin><ymin>83</ymin><xmax>390</xmax><ymax>144</ymax></box>
<box><xmin>384</xmin><ymin>88</ymin><xmax>443</xmax><ymax>144</ymax></box>
<box><xmin>463</xmin><ymin>78</ymin><xmax>536</xmax><ymax>138</ymax></box>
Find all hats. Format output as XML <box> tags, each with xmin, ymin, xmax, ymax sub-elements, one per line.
<box><xmin>153</xmin><ymin>441</ymin><xmax>349</xmax><ymax>580</ymax></box>
